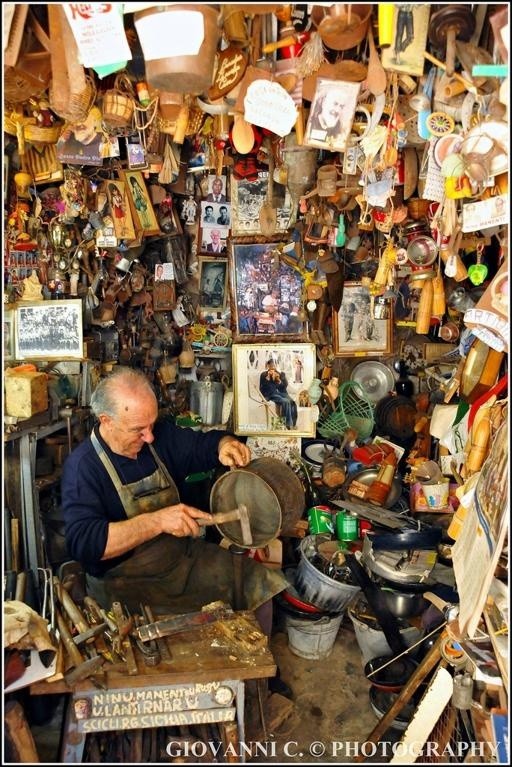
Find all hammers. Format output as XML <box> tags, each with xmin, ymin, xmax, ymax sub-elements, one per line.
<box><xmin>196</xmin><ymin>504</ymin><xmax>256</xmax><ymax>547</ymax></box>
<box><xmin>48</xmin><ymin>575</ymin><xmax>108</xmax><ymax>688</ymax></box>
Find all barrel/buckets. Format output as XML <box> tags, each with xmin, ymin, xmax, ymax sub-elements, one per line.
<box><xmin>189</xmin><ymin>376</ymin><xmax>224</xmax><ymax>426</ymax></box>
<box><xmin>283</xmin><ymin>612</ymin><xmax>343</xmax><ymax>660</ymax></box>
<box><xmin>452</xmin><ymin>672</ymin><xmax>473</xmax><ymax>709</ymax></box>
<box><xmin>210</xmin><ymin>458</ymin><xmax>306</xmax><ymax>549</ymax></box>
<box><xmin>133</xmin><ymin>6</ymin><xmax>220</xmax><ymax>99</ymax></box>
<box><xmin>348</xmin><ymin>609</ymin><xmax>428</xmax><ymax>670</ymax></box>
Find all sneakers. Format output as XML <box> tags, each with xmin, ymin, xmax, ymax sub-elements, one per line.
<box><xmin>268</xmin><ymin>665</ymin><xmax>292</xmax><ymax>697</ymax></box>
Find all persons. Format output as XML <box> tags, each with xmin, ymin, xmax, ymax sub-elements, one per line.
<box><xmin>393</xmin><ymin>274</ymin><xmax>412</xmax><ymax>319</ymax></box>
<box><xmin>8</xmin><ymin>250</ymin><xmax>41</xmax><ymax>286</ymax></box>
<box><xmin>203</xmin><ymin>206</ymin><xmax>215</xmax><ymax>223</ymax></box>
<box><xmin>130</xmin><ymin>176</ymin><xmax>150</xmax><ymax>227</ymax></box>
<box><xmin>207</xmin><ymin>178</ymin><xmax>226</xmax><ymax>204</ymax></box>
<box><xmin>293</xmin><ymin>357</ymin><xmax>303</xmax><ymax>384</ymax></box>
<box><xmin>491</xmin><ymin>196</ymin><xmax>505</xmax><ymax>216</ymax></box>
<box><xmin>311</xmin><ymin>91</ymin><xmax>345</xmax><ymax>142</ymax></box>
<box><xmin>62</xmin><ymin>368</ymin><xmax>292</xmax><ymax>701</ymax></box>
<box><xmin>217</xmin><ymin>206</ymin><xmax>230</xmax><ymax>225</ymax></box>
<box><xmin>58</xmin><ymin>119</ymin><xmax>102</xmax><ymax>165</ymax></box>
<box><xmin>464</xmin><ymin>204</ymin><xmax>480</xmax><ymax>225</ymax></box>
<box><xmin>249</xmin><ymin>351</ymin><xmax>255</xmax><ymax>366</ymax></box>
<box><xmin>203</xmin><ymin>278</ymin><xmax>212</xmax><ymax>304</ymax></box>
<box><xmin>358</xmin><ymin>298</ymin><xmax>376</xmax><ymax>341</ymax></box>
<box><xmin>155</xmin><ymin>265</ymin><xmax>164</xmax><ymax>281</ymax></box>
<box><xmin>207</xmin><ymin>228</ymin><xmax>226</xmax><ymax>254</ymax></box>
<box><xmin>18</xmin><ymin>307</ymin><xmax>80</xmax><ymax>351</ymax></box>
<box><xmin>108</xmin><ymin>183</ymin><xmax>128</xmax><ymax>236</ymax></box>
<box><xmin>393</xmin><ymin>4</ymin><xmax>420</xmax><ymax>65</ymax></box>
<box><xmin>259</xmin><ymin>359</ymin><xmax>298</xmax><ymax>430</ymax></box>
<box><xmin>234</xmin><ymin>218</ymin><xmax>291</xmax><ymax>232</ymax></box>
<box><xmin>215</xmin><ymin>279</ymin><xmax>224</xmax><ymax>305</ymax></box>
<box><xmin>340</xmin><ymin>296</ymin><xmax>358</xmax><ymax>342</ymax></box>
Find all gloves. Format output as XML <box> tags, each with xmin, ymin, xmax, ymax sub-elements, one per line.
<box><xmin>49</xmin><ymin>67</ymin><xmax>95</xmax><ymax>122</ymax></box>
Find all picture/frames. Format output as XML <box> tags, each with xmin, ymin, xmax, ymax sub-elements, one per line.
<box><xmin>3</xmin><ymin>298</ymin><xmax>87</xmax><ymax>367</ymax></box>
<box><xmin>187</xmin><ymin>170</ymin><xmax>393</xmax><ymax>438</ymax></box>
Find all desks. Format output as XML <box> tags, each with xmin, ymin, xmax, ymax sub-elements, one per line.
<box><xmin>29</xmin><ymin>608</ymin><xmax>278</xmax><ymax>762</ymax></box>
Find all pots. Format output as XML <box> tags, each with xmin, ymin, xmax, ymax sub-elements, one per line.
<box><xmin>364</xmin><ymin>654</ymin><xmax>420</xmax><ymax>693</ymax></box>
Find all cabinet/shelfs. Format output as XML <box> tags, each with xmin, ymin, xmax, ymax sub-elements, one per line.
<box><xmin>19</xmin><ymin>408</ymin><xmax>96</xmax><ymax>590</ymax></box>
